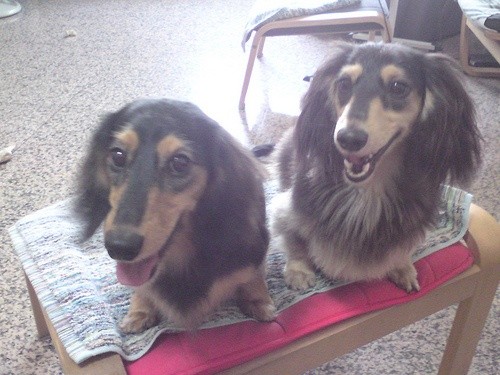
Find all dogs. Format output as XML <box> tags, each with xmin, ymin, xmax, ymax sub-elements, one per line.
<box><xmin>269</xmin><ymin>41</ymin><xmax>486</xmax><ymax>293</ymax></box>
<box><xmin>65</xmin><ymin>100</ymin><xmax>280</xmax><ymax>336</ymax></box>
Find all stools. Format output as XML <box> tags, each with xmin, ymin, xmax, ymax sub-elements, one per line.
<box><xmin>9</xmin><ymin>153</ymin><xmax>500</xmax><ymax>375</ymax></box>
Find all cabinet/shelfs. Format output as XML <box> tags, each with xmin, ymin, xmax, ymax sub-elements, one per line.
<box><xmin>458</xmin><ymin>0</ymin><xmax>500</xmax><ymax>78</ymax></box>
<box><xmin>238</xmin><ymin>0</ymin><xmax>393</xmax><ymax>110</ymax></box>
